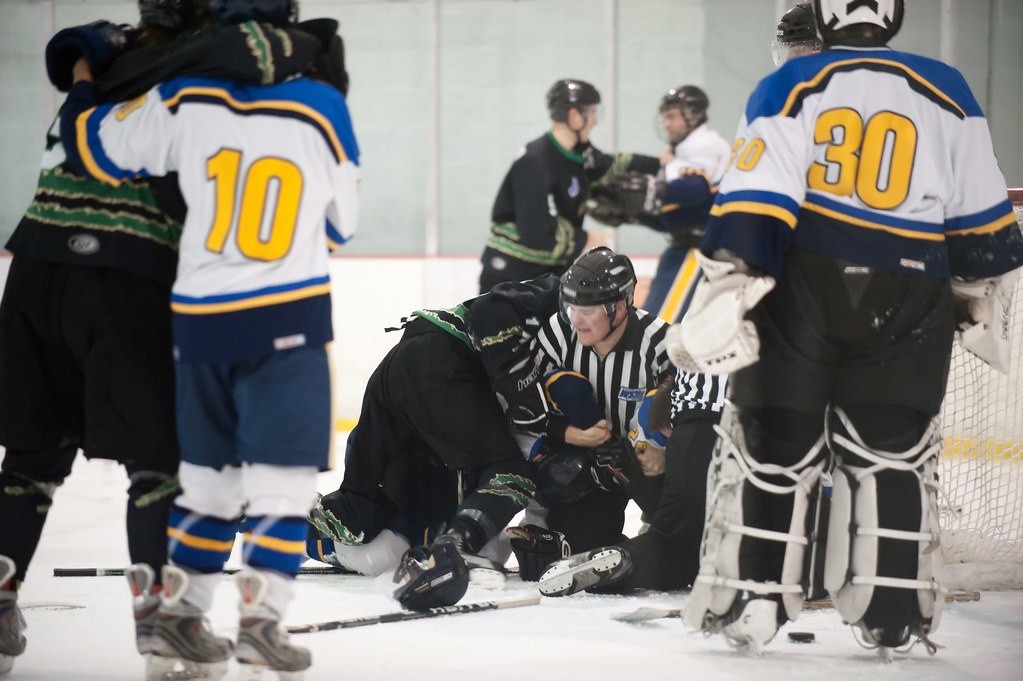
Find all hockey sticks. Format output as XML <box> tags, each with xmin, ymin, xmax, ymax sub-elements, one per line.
<box><xmin>282</xmin><ymin>591</ymin><xmax>539</xmax><ymax>635</ymax></box>
<box><xmin>610</xmin><ymin>590</ymin><xmax>980</xmax><ymax>623</ymax></box>
<box><xmin>52</xmin><ymin>564</ymin><xmax>363</xmax><ymax>578</ymax></box>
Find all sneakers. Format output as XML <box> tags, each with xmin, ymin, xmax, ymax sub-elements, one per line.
<box><xmin>146</xmin><ymin>565</ymin><xmax>236</xmax><ymax>681</ymax></box>
<box><xmin>538</xmin><ymin>547</ymin><xmax>632</xmax><ymax>598</ymax></box>
<box><xmin>127</xmin><ymin>563</ymin><xmax>161</xmax><ymax>668</ymax></box>
<box><xmin>0</xmin><ymin>554</ymin><xmax>26</xmax><ymax>674</ymax></box>
<box><xmin>233</xmin><ymin>569</ymin><xmax>312</xmax><ymax>681</ymax></box>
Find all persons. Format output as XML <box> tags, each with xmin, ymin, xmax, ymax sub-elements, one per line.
<box><xmin>479</xmin><ymin>79</ymin><xmax>668</xmax><ymax>296</ymax></box>
<box><xmin>588</xmin><ymin>84</ymin><xmax>732</xmax><ymax>314</ymax></box>
<box><xmin>302</xmin><ymin>268</ymin><xmax>613</xmax><ymax>612</ymax></box>
<box><xmin>665</xmin><ymin>0</ymin><xmax>1023</xmax><ymax>665</ymax></box>
<box><xmin>530</xmin><ymin>247</ymin><xmax>731</xmax><ymax>598</ymax></box>
<box><xmin>0</xmin><ymin>0</ymin><xmax>348</xmax><ymax>681</ymax></box>
<box><xmin>58</xmin><ymin>0</ymin><xmax>361</xmax><ymax>681</ymax></box>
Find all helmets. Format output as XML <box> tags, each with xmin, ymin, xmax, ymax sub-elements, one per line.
<box><xmin>217</xmin><ymin>0</ymin><xmax>294</xmax><ymax>25</ymax></box>
<box><xmin>392</xmin><ymin>544</ymin><xmax>469</xmax><ymax>614</ymax></box>
<box><xmin>813</xmin><ymin>0</ymin><xmax>905</xmax><ymax>42</ymax></box>
<box><xmin>558</xmin><ymin>246</ymin><xmax>637</xmax><ymax>306</ymax></box>
<box><xmin>548</xmin><ymin>80</ymin><xmax>600</xmax><ymax>120</ymax></box>
<box><xmin>772</xmin><ymin>0</ymin><xmax>819</xmax><ymax>69</ymax></box>
<box><xmin>659</xmin><ymin>85</ymin><xmax>708</xmax><ymax>112</ymax></box>
<box><xmin>138</xmin><ymin>0</ymin><xmax>216</xmax><ymax>31</ymax></box>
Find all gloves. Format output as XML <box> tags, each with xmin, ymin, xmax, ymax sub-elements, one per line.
<box><xmin>591</xmin><ymin>454</ymin><xmax>637</xmax><ymax>495</ymax></box>
<box><xmin>46</xmin><ymin>16</ymin><xmax>139</xmax><ymax>90</ymax></box>
<box><xmin>302</xmin><ymin>16</ymin><xmax>350</xmax><ymax>98</ymax></box>
<box><xmin>586</xmin><ymin>149</ymin><xmax>663</xmax><ymax>226</ymax></box>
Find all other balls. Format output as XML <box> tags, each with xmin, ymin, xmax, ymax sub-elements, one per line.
<box><xmin>786</xmin><ymin>632</ymin><xmax>816</xmax><ymax>645</ymax></box>
